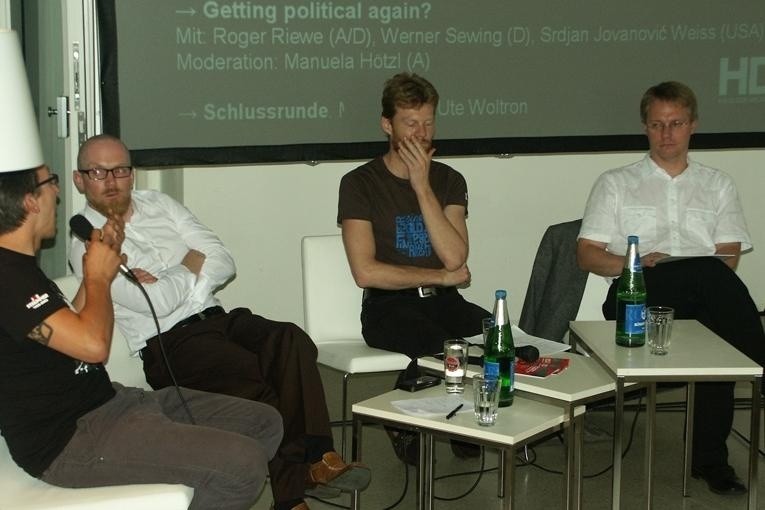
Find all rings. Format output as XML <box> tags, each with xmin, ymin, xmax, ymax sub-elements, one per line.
<box><xmin>96</xmin><ymin>228</ymin><xmax>104</xmax><ymax>241</ymax></box>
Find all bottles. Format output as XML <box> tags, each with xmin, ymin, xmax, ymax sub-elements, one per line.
<box><xmin>617</xmin><ymin>236</ymin><xmax>648</xmax><ymax>348</ymax></box>
<box><xmin>483</xmin><ymin>290</ymin><xmax>518</xmax><ymax>407</ymax></box>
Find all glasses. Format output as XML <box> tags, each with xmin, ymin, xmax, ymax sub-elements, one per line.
<box><xmin>645</xmin><ymin>118</ymin><xmax>690</xmax><ymax>129</ymax></box>
<box><xmin>77</xmin><ymin>164</ymin><xmax>134</xmax><ymax>182</ymax></box>
<box><xmin>36</xmin><ymin>172</ymin><xmax>60</xmax><ymax>187</ymax></box>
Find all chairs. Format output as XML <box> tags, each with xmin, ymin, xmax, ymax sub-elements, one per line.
<box><xmin>519</xmin><ymin>218</ymin><xmax>697</xmax><ymax>497</ymax></box>
<box><xmin>300</xmin><ymin>232</ymin><xmax>417</xmax><ymax>491</ymax></box>
<box><xmin>0</xmin><ymin>272</ymin><xmax>196</xmax><ymax>510</ymax></box>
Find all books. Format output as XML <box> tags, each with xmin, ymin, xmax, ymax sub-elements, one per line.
<box><xmin>513</xmin><ymin>356</ymin><xmax>571</xmax><ymax>379</ymax></box>
<box><xmin>389</xmin><ymin>394</ymin><xmax>476</xmax><ymax>417</ymax></box>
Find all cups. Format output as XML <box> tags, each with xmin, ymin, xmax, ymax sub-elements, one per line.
<box><xmin>481</xmin><ymin>316</ymin><xmax>497</xmax><ymax>348</ymax></box>
<box><xmin>647</xmin><ymin>306</ymin><xmax>676</xmax><ymax>356</ymax></box>
<box><xmin>470</xmin><ymin>374</ymin><xmax>503</xmax><ymax>427</ymax></box>
<box><xmin>442</xmin><ymin>340</ymin><xmax>471</xmax><ymax>394</ymax></box>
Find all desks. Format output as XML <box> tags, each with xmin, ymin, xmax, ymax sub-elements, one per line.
<box><xmin>415</xmin><ymin>331</ymin><xmax>658</xmax><ymax>510</ymax></box>
<box><xmin>348</xmin><ymin>372</ymin><xmax>589</xmax><ymax>510</ymax></box>
<box><xmin>567</xmin><ymin>321</ymin><xmax>764</xmax><ymax>509</ymax></box>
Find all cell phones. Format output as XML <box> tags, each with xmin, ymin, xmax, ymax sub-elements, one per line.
<box><xmin>399</xmin><ymin>374</ymin><xmax>441</xmax><ymax>392</ymax></box>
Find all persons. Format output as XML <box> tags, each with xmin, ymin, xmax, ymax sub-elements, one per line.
<box><xmin>0</xmin><ymin>163</ymin><xmax>287</xmax><ymax>510</ymax></box>
<box><xmin>64</xmin><ymin>133</ymin><xmax>372</xmax><ymax>508</ymax></box>
<box><xmin>334</xmin><ymin>69</ymin><xmax>498</xmax><ymax>471</ymax></box>
<box><xmin>573</xmin><ymin>78</ymin><xmax>765</xmax><ymax>495</ymax></box>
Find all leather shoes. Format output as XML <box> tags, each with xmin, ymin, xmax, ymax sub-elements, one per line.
<box><xmin>307</xmin><ymin>447</ymin><xmax>374</xmax><ymax>500</ymax></box>
<box><xmin>694</xmin><ymin>466</ymin><xmax>746</xmax><ymax>496</ymax></box>
<box><xmin>451</xmin><ymin>438</ymin><xmax>480</xmax><ymax>459</ymax></box>
<box><xmin>386</xmin><ymin>429</ymin><xmax>436</xmax><ymax>465</ymax></box>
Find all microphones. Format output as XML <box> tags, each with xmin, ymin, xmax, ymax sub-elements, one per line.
<box><xmin>67</xmin><ymin>211</ymin><xmax>142</xmax><ymax>284</ymax></box>
<box><xmin>435</xmin><ymin>352</ymin><xmax>511</xmax><ymax>371</ymax></box>
<box><xmin>478</xmin><ymin>344</ymin><xmax>540</xmax><ymax>362</ymax></box>
